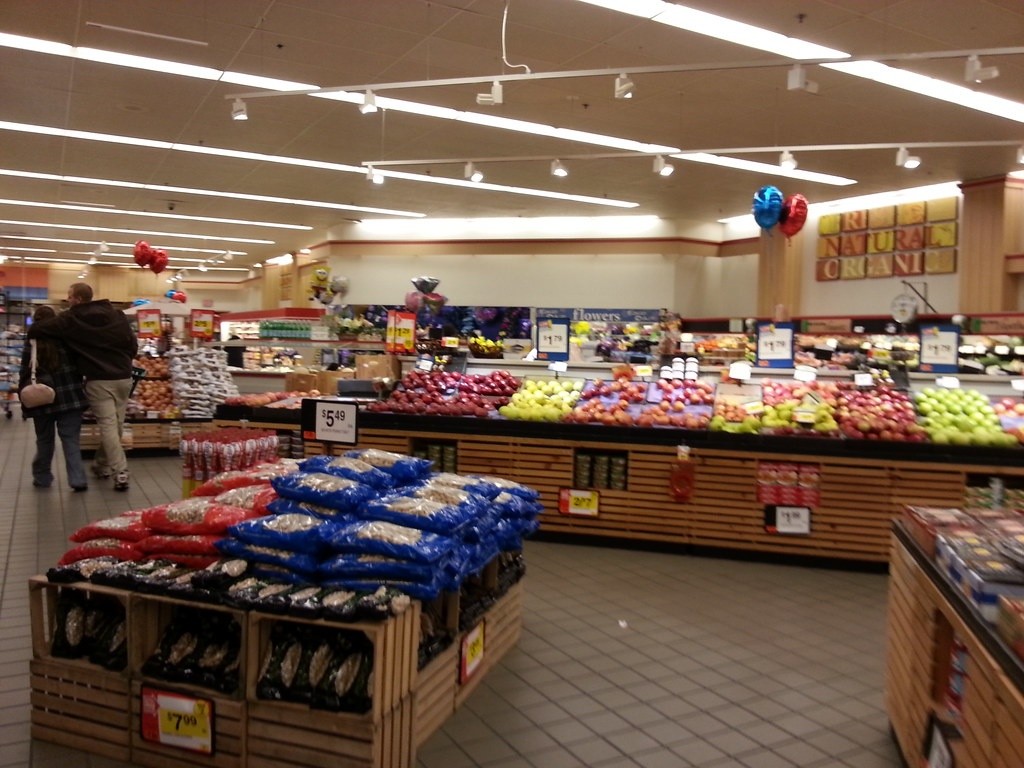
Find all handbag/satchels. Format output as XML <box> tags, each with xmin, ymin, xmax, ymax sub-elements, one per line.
<box><xmin>18</xmin><ymin>338</ymin><xmax>56</xmax><ymax>408</ymax></box>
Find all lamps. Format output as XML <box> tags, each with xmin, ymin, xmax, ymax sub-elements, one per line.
<box><xmin>0</xmin><ymin>42</ymin><xmax>1023</xmax><ymax>287</ymax></box>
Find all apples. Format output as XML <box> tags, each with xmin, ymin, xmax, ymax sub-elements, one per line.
<box><xmin>366</xmin><ymin>367</ymin><xmax>1024</xmax><ymax>450</ymax></box>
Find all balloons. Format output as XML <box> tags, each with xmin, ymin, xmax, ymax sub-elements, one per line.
<box><xmin>461</xmin><ymin>306</ymin><xmax>531</xmax><ymax>339</ymax></box>
<box><xmin>150</xmin><ymin>248</ymin><xmax>168</xmax><ymax>277</ymax></box>
<box><xmin>164</xmin><ymin>289</ymin><xmax>187</xmax><ymax>303</ymax></box>
<box><xmin>135</xmin><ymin>240</ymin><xmax>151</xmax><ymax>270</ymax></box>
<box><xmin>404</xmin><ymin>274</ymin><xmax>444</xmax><ymax>330</ymax></box>
<box><xmin>752</xmin><ymin>185</ymin><xmax>785</xmax><ymax>237</ymax></box>
<box><xmin>777</xmin><ymin>192</ymin><xmax>808</xmax><ymax>242</ymax></box>
<box><xmin>304</xmin><ymin>262</ymin><xmax>348</xmax><ymax>307</ymax></box>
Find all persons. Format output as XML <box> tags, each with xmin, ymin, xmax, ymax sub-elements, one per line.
<box><xmin>19</xmin><ymin>304</ymin><xmax>89</xmax><ymax>493</ymax></box>
<box><xmin>28</xmin><ymin>282</ymin><xmax>138</xmax><ymax>491</ymax></box>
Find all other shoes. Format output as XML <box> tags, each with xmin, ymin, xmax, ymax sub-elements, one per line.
<box><xmin>115</xmin><ymin>482</ymin><xmax>130</xmax><ymax>490</ymax></box>
<box><xmin>73</xmin><ymin>486</ymin><xmax>89</xmax><ymax>492</ymax></box>
<box><xmin>89</xmin><ymin>464</ymin><xmax>109</xmax><ymax>478</ymax></box>
<box><xmin>32</xmin><ymin>479</ymin><xmax>52</xmax><ymax>487</ymax></box>
